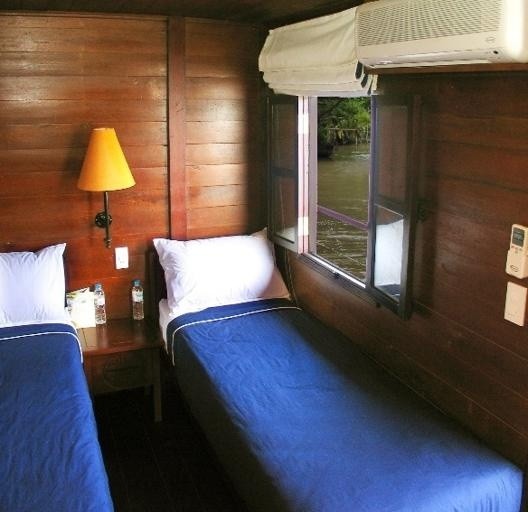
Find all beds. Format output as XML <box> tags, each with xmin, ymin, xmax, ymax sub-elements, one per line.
<box><xmin>145</xmin><ymin>248</ymin><xmax>522</xmax><ymax>512</ymax></box>
<box><xmin>0</xmin><ymin>254</ymin><xmax>115</xmax><ymax>512</ymax></box>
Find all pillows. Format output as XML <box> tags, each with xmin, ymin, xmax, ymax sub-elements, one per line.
<box><xmin>153</xmin><ymin>225</ymin><xmax>292</xmax><ymax>316</ymax></box>
<box><xmin>1</xmin><ymin>242</ymin><xmax>68</xmax><ymax>327</ymax></box>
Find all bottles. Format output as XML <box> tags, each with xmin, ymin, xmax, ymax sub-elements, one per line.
<box><xmin>94</xmin><ymin>284</ymin><xmax>107</xmax><ymax>324</ymax></box>
<box><xmin>130</xmin><ymin>280</ymin><xmax>145</xmax><ymax>321</ymax></box>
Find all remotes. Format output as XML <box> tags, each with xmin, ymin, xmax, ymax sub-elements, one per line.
<box><xmin>510</xmin><ymin>223</ymin><xmax>528</xmax><ymax>256</ymax></box>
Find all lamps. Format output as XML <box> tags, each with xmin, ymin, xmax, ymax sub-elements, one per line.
<box><xmin>77</xmin><ymin>127</ymin><xmax>136</xmax><ymax>249</ymax></box>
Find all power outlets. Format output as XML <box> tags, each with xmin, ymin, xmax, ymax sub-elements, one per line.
<box><xmin>115</xmin><ymin>246</ymin><xmax>129</xmax><ymax>270</ymax></box>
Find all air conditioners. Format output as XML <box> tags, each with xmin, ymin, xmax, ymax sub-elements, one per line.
<box><xmin>354</xmin><ymin>0</ymin><xmax>528</xmax><ymax>75</ymax></box>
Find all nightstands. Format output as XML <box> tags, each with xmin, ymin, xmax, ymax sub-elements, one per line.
<box><xmin>77</xmin><ymin>317</ymin><xmax>164</xmax><ymax>427</ymax></box>
